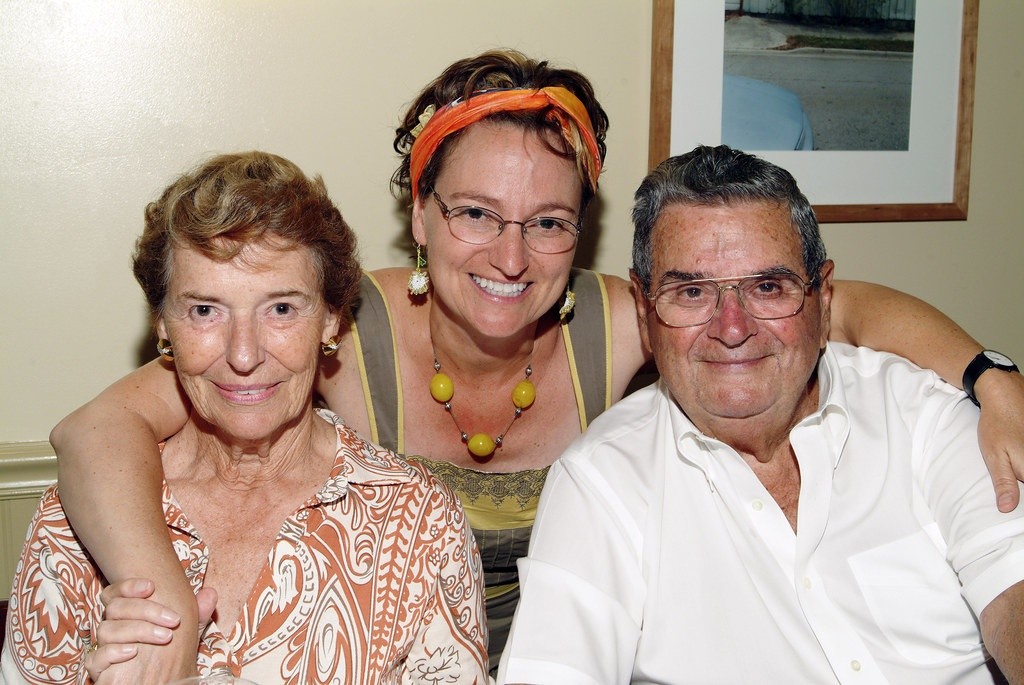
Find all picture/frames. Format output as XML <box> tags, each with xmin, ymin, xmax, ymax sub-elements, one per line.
<box><xmin>649</xmin><ymin>0</ymin><xmax>981</xmax><ymax>225</ymax></box>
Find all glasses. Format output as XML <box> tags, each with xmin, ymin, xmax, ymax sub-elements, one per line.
<box><xmin>429</xmin><ymin>185</ymin><xmax>581</xmax><ymax>255</ymax></box>
<box><xmin>641</xmin><ymin>270</ymin><xmax>815</xmax><ymax>328</ymax></box>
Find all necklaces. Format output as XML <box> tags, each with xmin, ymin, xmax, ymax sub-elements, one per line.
<box><xmin>430</xmin><ymin>314</ymin><xmax>536</xmax><ymax>456</ymax></box>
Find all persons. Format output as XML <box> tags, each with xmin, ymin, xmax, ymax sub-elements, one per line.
<box><xmin>496</xmin><ymin>147</ymin><xmax>1024</xmax><ymax>685</ymax></box>
<box><xmin>0</xmin><ymin>150</ymin><xmax>494</xmax><ymax>685</ymax></box>
<box><xmin>49</xmin><ymin>50</ymin><xmax>1024</xmax><ymax>685</ymax></box>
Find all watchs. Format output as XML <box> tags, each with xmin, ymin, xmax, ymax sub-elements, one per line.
<box><xmin>962</xmin><ymin>350</ymin><xmax>1020</xmax><ymax>407</ymax></box>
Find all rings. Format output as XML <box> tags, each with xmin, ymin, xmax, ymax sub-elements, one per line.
<box><xmin>91</xmin><ymin>642</ymin><xmax>97</xmax><ymax>650</ymax></box>
